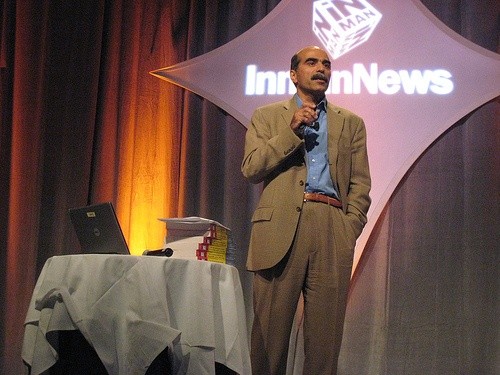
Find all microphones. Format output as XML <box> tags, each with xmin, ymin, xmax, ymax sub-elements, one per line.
<box><xmin>143</xmin><ymin>247</ymin><xmax>173</xmax><ymax>257</ymax></box>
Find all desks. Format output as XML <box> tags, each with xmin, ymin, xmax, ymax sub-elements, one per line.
<box><xmin>21</xmin><ymin>253</ymin><xmax>252</xmax><ymax>375</ymax></box>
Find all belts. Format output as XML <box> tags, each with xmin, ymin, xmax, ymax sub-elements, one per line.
<box><xmin>303</xmin><ymin>192</ymin><xmax>342</xmax><ymax>209</ymax></box>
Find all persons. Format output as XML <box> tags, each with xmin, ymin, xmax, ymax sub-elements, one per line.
<box><xmin>240</xmin><ymin>46</ymin><xmax>371</xmax><ymax>375</ymax></box>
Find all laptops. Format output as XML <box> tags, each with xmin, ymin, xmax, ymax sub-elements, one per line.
<box><xmin>69</xmin><ymin>202</ymin><xmax>131</xmax><ymax>256</ymax></box>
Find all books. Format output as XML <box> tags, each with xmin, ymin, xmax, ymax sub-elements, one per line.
<box><xmin>162</xmin><ymin>215</ymin><xmax>230</xmax><ymax>264</ymax></box>
